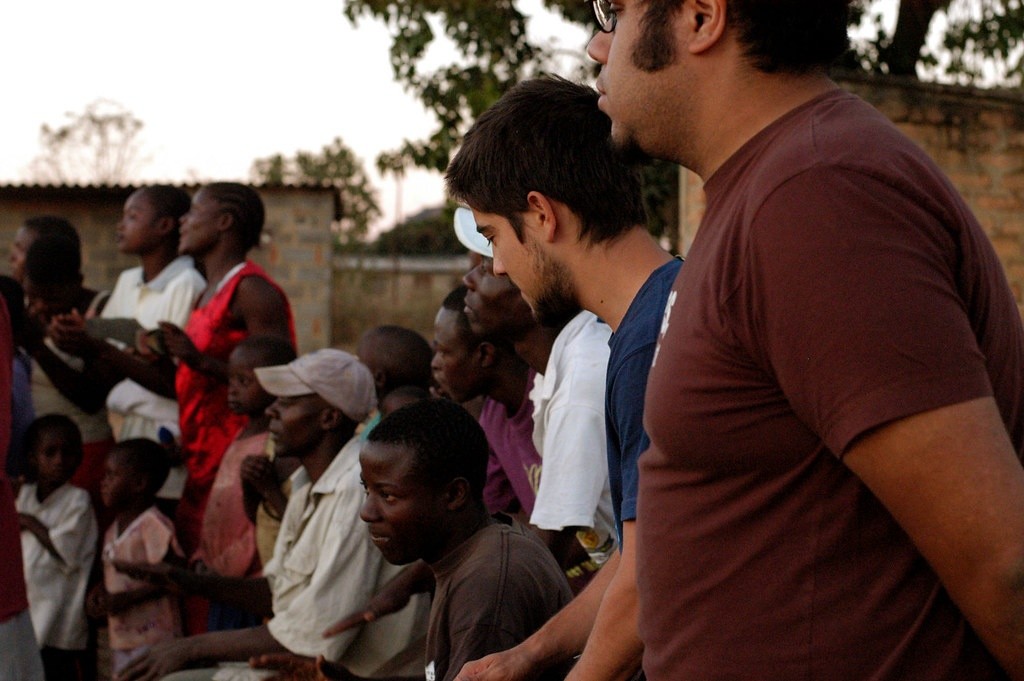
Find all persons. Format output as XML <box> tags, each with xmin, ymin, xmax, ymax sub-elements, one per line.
<box><xmin>0</xmin><ymin>182</ymin><xmax>621</xmax><ymax>680</ymax></box>
<box><xmin>587</xmin><ymin>0</ymin><xmax>1024</xmax><ymax>681</ymax></box>
<box><xmin>444</xmin><ymin>71</ymin><xmax>687</xmax><ymax>681</ymax></box>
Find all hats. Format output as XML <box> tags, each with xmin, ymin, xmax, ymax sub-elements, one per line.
<box><xmin>453</xmin><ymin>208</ymin><xmax>494</xmax><ymax>257</ymax></box>
<box><xmin>253</xmin><ymin>348</ymin><xmax>376</xmax><ymax>421</ymax></box>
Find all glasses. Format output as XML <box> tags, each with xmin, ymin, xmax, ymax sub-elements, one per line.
<box><xmin>590</xmin><ymin>0</ymin><xmax>617</xmax><ymax>33</ymax></box>
